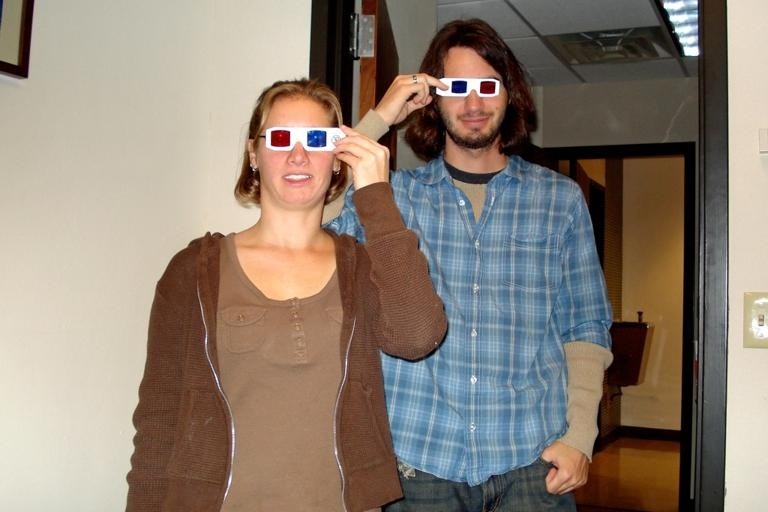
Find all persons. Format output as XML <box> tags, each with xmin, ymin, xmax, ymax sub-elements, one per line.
<box><xmin>323</xmin><ymin>21</ymin><xmax>615</xmax><ymax>512</ymax></box>
<box><xmin>124</xmin><ymin>77</ymin><xmax>448</xmax><ymax>512</ymax></box>
